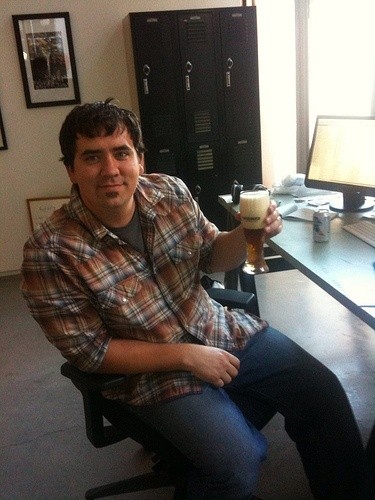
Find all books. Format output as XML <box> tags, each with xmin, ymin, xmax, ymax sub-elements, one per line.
<box><xmin>274</xmin><ymin>204</ymin><xmax>339</xmax><ymax>222</ymax></box>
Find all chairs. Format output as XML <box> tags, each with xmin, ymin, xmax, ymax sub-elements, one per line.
<box><xmin>59</xmin><ymin>275</ymin><xmax>278</xmax><ymax>500</ymax></box>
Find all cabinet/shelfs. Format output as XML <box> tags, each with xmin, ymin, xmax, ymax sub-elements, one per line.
<box><xmin>123</xmin><ymin>5</ymin><xmax>263</xmax><ymax>233</ymax></box>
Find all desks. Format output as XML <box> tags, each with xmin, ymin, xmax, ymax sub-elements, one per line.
<box><xmin>218</xmin><ymin>186</ymin><xmax>375</xmax><ymax>331</ymax></box>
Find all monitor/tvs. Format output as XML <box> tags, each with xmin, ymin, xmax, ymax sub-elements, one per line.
<box><xmin>303</xmin><ymin>114</ymin><xmax>375</xmax><ymax>211</ymax></box>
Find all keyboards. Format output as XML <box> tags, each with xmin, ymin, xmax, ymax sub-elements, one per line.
<box><xmin>342</xmin><ymin>220</ymin><xmax>375</xmax><ymax>247</ymax></box>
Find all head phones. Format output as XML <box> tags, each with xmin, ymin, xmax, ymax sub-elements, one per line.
<box><xmin>230</xmin><ymin>183</ymin><xmax>271</xmax><ymax>204</ymax></box>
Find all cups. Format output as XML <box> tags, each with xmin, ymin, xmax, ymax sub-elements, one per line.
<box><xmin>240</xmin><ymin>190</ymin><xmax>270</xmax><ymax>275</ymax></box>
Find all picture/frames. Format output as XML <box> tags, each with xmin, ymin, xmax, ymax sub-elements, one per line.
<box><xmin>11</xmin><ymin>12</ymin><xmax>82</xmax><ymax>110</ymax></box>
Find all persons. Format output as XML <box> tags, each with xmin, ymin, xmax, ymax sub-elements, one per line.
<box><xmin>21</xmin><ymin>96</ymin><xmax>375</xmax><ymax>500</ymax></box>
<box><xmin>31</xmin><ymin>36</ymin><xmax>69</xmax><ymax>89</ymax></box>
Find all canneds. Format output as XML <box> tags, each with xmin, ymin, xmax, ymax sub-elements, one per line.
<box><xmin>313</xmin><ymin>209</ymin><xmax>330</xmax><ymax>242</ymax></box>
